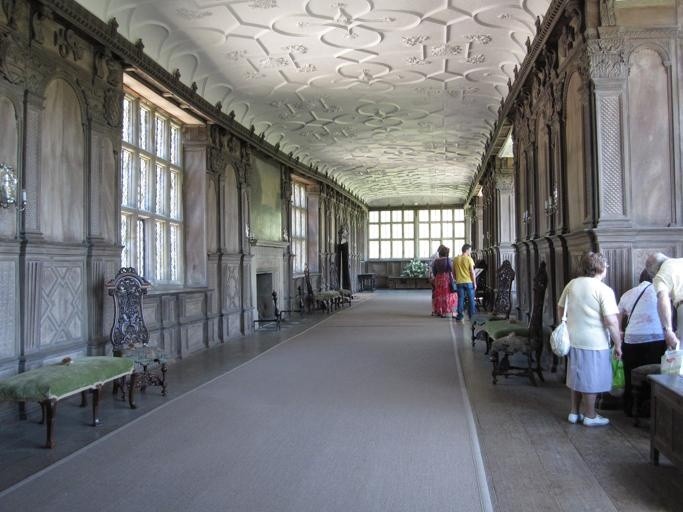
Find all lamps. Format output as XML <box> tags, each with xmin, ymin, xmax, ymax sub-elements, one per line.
<box><xmin>0</xmin><ymin>163</ymin><xmax>28</xmax><ymax>214</ymax></box>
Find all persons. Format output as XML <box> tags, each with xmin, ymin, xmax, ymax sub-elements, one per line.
<box><xmin>646</xmin><ymin>251</ymin><xmax>683</xmax><ymax>352</ymax></box>
<box><xmin>557</xmin><ymin>252</ymin><xmax>623</xmax><ymax>428</ymax></box>
<box><xmin>428</xmin><ymin>243</ymin><xmax>477</xmax><ymax>321</ymax></box>
<box><xmin>616</xmin><ymin>267</ymin><xmax>680</xmax><ymax>418</ymax></box>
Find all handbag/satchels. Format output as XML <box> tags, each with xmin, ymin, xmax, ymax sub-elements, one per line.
<box><xmin>548</xmin><ymin>321</ymin><xmax>571</xmax><ymax>357</ymax></box>
<box><xmin>609</xmin><ymin>344</ymin><xmax>626</xmax><ymax>389</ymax></box>
<box><xmin>451</xmin><ymin>280</ymin><xmax>457</xmax><ymax>292</ymax></box>
<box><xmin>659</xmin><ymin>343</ymin><xmax>683</xmax><ymax>377</ymax></box>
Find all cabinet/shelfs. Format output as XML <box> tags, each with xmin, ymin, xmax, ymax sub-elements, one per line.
<box><xmin>648</xmin><ymin>373</ymin><xmax>683</xmax><ymax>471</ymax></box>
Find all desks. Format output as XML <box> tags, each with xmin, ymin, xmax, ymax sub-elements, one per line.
<box><xmin>358</xmin><ymin>273</ymin><xmax>376</xmax><ymax>291</ymax></box>
<box><xmin>386</xmin><ymin>274</ymin><xmax>433</xmax><ymax>290</ymax></box>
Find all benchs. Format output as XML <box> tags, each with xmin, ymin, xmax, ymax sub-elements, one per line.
<box><xmin>1</xmin><ymin>356</ymin><xmax>138</xmax><ymax>448</ymax></box>
<box><xmin>476</xmin><ymin>320</ymin><xmax>534</xmax><ymax>363</ymax></box>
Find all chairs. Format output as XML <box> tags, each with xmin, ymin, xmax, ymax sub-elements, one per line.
<box><xmin>490</xmin><ymin>261</ymin><xmax>548</xmax><ymax>387</ymax></box>
<box><xmin>105</xmin><ymin>266</ymin><xmax>168</xmax><ymax>408</ymax></box>
<box><xmin>464</xmin><ymin>259</ymin><xmax>490</xmax><ymax>311</ymax></box>
<box><xmin>329</xmin><ymin>266</ymin><xmax>352</xmax><ymax>310</ymax></box>
<box><xmin>472</xmin><ymin>260</ymin><xmax>516</xmax><ymax>355</ymax></box>
<box><xmin>304</xmin><ymin>268</ymin><xmax>333</xmax><ymax>314</ymax></box>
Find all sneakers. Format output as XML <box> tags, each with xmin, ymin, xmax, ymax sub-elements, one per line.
<box><xmin>567</xmin><ymin>413</ymin><xmax>578</xmax><ymax>424</ymax></box>
<box><xmin>582</xmin><ymin>415</ymin><xmax>609</xmax><ymax>427</ymax></box>
